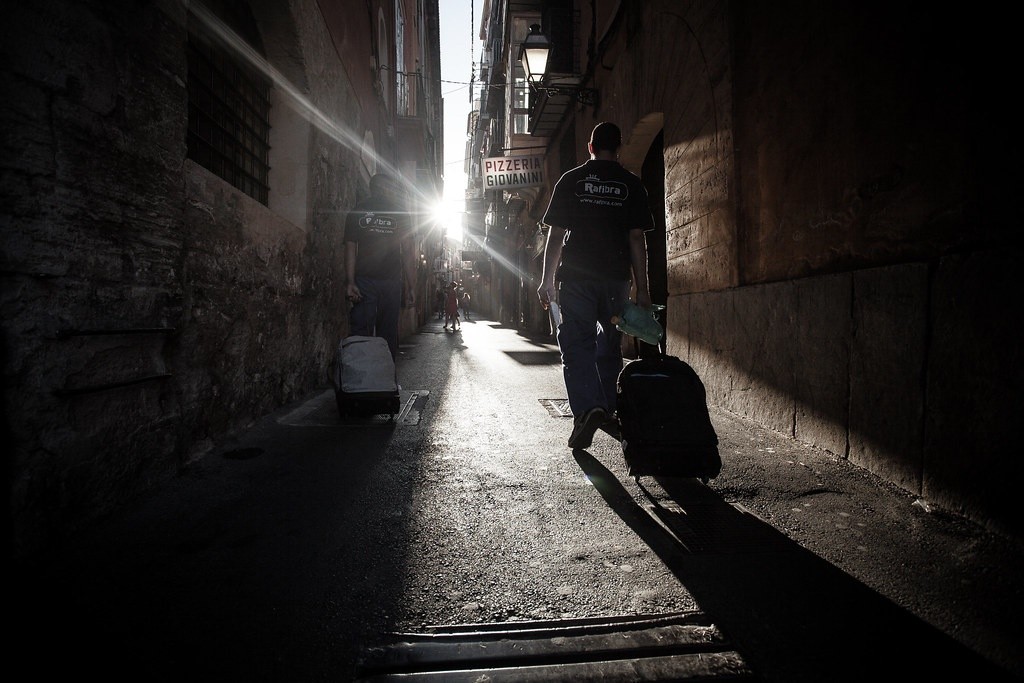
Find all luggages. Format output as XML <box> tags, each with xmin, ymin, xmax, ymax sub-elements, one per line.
<box><xmin>617</xmin><ymin>336</ymin><xmax>723</xmax><ymax>485</ymax></box>
<box><xmin>330</xmin><ymin>298</ymin><xmax>401</xmax><ymax>418</ymax></box>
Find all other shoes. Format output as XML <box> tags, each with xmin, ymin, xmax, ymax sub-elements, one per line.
<box><xmin>568</xmin><ymin>409</ymin><xmax>606</xmax><ymax>450</ymax></box>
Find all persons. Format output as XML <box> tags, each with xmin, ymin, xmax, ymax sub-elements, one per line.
<box><xmin>537</xmin><ymin>121</ymin><xmax>656</xmax><ymax>448</ymax></box>
<box><xmin>436</xmin><ymin>280</ymin><xmax>470</xmax><ymax>328</ymax></box>
<box><xmin>342</xmin><ymin>172</ymin><xmax>417</xmax><ymax>364</ymax></box>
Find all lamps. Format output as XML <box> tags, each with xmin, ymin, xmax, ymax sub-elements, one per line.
<box><xmin>517</xmin><ymin>23</ymin><xmax>602</xmax><ymax>117</ymax></box>
